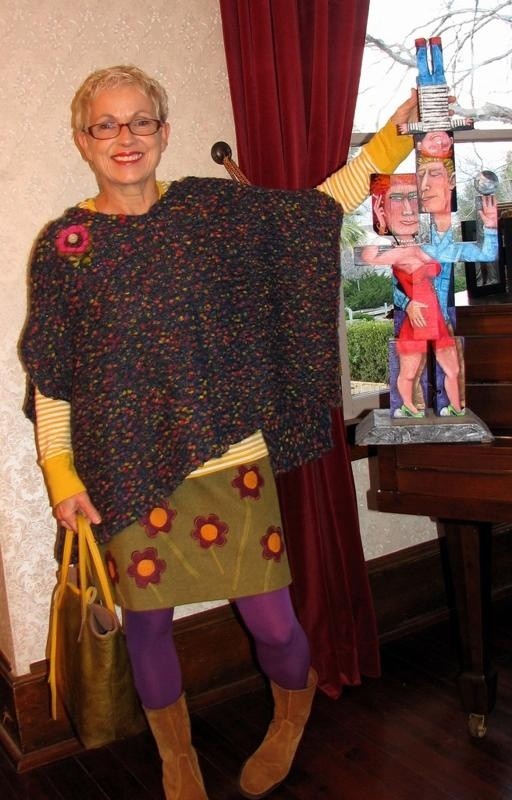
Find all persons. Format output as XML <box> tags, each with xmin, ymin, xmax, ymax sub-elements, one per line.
<box><xmin>24</xmin><ymin>66</ymin><xmax>454</xmax><ymax>799</ymax></box>
<box><xmin>359</xmin><ymin>176</ymin><xmax>464</xmax><ymax>418</ymax></box>
<box><xmin>385</xmin><ymin>159</ymin><xmax>499</xmax><ymax>415</ymax></box>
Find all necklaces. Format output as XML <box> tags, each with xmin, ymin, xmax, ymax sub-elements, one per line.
<box><xmin>393</xmin><ymin>238</ymin><xmax>417</xmax><ymax>248</ymax></box>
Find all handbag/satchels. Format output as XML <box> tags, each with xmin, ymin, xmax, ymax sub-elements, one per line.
<box><xmin>47</xmin><ymin>567</ymin><xmax>136</xmax><ymax>750</ymax></box>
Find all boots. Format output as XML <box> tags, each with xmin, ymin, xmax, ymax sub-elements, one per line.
<box><xmin>240</xmin><ymin>668</ymin><xmax>319</xmax><ymax>799</ymax></box>
<box><xmin>143</xmin><ymin>690</ymin><xmax>208</xmax><ymax>800</ymax></box>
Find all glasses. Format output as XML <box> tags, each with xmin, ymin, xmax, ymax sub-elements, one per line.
<box><xmin>82</xmin><ymin>119</ymin><xmax>163</xmax><ymax>140</ymax></box>
